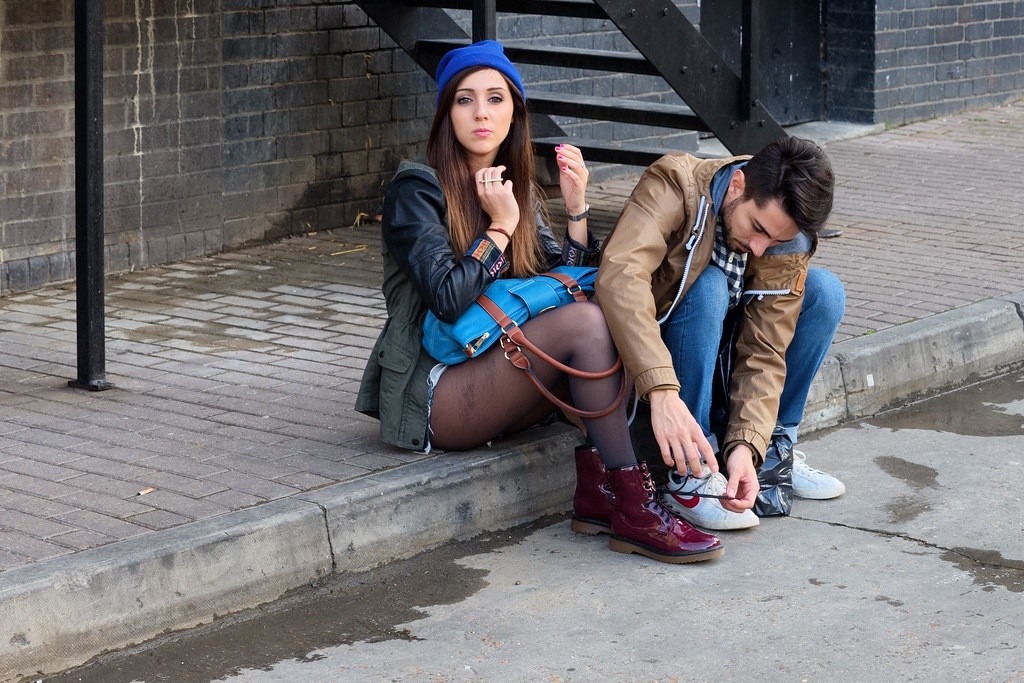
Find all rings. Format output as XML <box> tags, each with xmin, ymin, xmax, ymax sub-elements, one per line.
<box><xmin>478</xmin><ymin>173</ymin><xmax>503</xmax><ymax>190</ymax></box>
<box><xmin>581</xmin><ymin>161</ymin><xmax>585</xmax><ymax>168</ymax></box>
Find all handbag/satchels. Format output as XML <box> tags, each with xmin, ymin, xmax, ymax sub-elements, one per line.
<box><xmin>421</xmin><ymin>264</ymin><xmax>627</xmax><ymax>419</ymax></box>
<box><xmin>745</xmin><ymin>418</ymin><xmax>795</xmax><ymax>519</ymax></box>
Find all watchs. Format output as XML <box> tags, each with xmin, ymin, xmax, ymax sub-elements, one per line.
<box><xmin>566</xmin><ymin>204</ymin><xmax>590</xmax><ymax>221</ymax></box>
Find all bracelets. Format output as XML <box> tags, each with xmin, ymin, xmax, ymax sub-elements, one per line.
<box><xmin>486</xmin><ymin>228</ymin><xmax>512</xmax><ymax>242</ymax></box>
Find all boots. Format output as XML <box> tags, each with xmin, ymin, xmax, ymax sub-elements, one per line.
<box><xmin>570</xmin><ymin>444</ymin><xmax>613</xmax><ymax>535</ymax></box>
<box><xmin>599</xmin><ymin>460</ymin><xmax>725</xmax><ymax>564</ymax></box>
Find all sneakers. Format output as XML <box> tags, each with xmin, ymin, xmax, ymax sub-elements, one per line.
<box><xmin>790</xmin><ymin>449</ymin><xmax>845</xmax><ymax>500</ymax></box>
<box><xmin>648</xmin><ymin>461</ymin><xmax>760</xmax><ymax>530</ymax></box>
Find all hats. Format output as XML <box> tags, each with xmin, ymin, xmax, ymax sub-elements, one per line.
<box><xmin>434</xmin><ymin>39</ymin><xmax>527</xmax><ymax>105</ymax></box>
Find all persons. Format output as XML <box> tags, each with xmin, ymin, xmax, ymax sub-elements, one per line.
<box><xmin>592</xmin><ymin>138</ymin><xmax>845</xmax><ymax>530</ymax></box>
<box><xmin>354</xmin><ymin>40</ymin><xmax>725</xmax><ymax>563</ymax></box>
